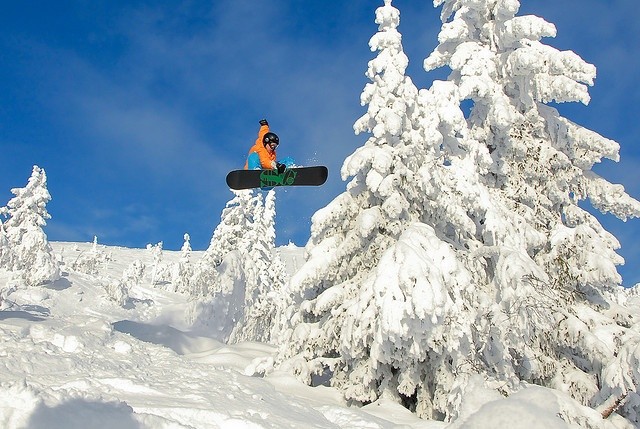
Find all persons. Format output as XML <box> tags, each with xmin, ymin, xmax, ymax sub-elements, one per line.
<box><xmin>244</xmin><ymin>119</ymin><xmax>286</xmax><ymax>174</ymax></box>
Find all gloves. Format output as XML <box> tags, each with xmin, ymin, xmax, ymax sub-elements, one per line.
<box><xmin>259</xmin><ymin>119</ymin><xmax>268</xmax><ymax>126</ymax></box>
<box><xmin>276</xmin><ymin>163</ymin><xmax>286</xmax><ymax>173</ymax></box>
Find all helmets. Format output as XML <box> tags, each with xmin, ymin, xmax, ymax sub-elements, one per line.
<box><xmin>263</xmin><ymin>132</ymin><xmax>279</xmax><ymax>144</ymax></box>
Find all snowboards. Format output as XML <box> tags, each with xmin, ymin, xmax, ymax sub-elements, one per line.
<box><xmin>224</xmin><ymin>166</ymin><xmax>330</xmax><ymax>190</ymax></box>
